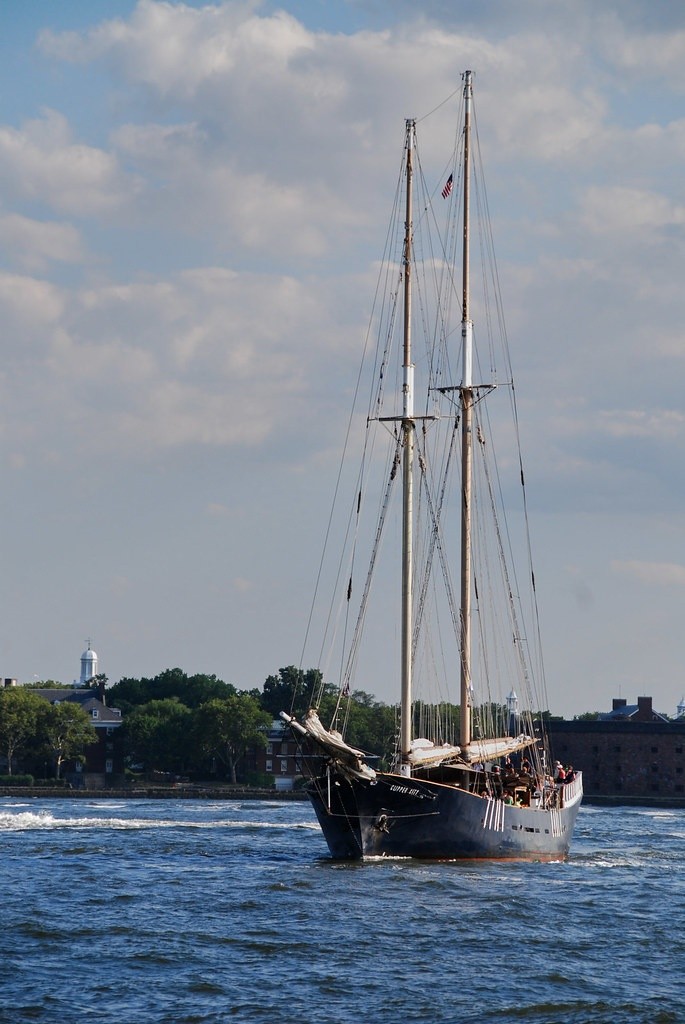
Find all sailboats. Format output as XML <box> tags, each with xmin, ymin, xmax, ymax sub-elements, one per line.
<box><xmin>274</xmin><ymin>72</ymin><xmax>586</xmax><ymax>864</ymax></box>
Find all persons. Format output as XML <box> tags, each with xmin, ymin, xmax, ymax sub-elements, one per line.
<box><xmin>474</xmin><ymin>758</ymin><xmax>575</xmax><ymax>805</ymax></box>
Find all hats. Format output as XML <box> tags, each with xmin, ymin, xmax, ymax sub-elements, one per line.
<box><xmin>557</xmin><ymin>765</ymin><xmax>563</xmax><ymax>768</ymax></box>
<box><xmin>553</xmin><ymin>760</ymin><xmax>560</xmax><ymax>764</ymax></box>
